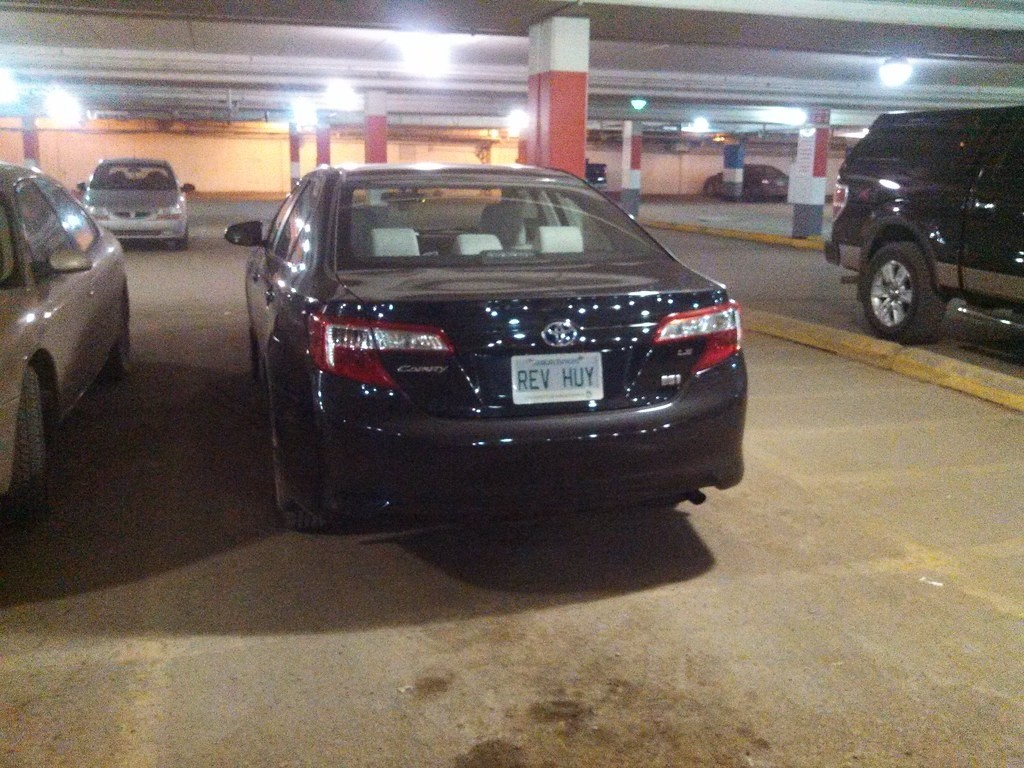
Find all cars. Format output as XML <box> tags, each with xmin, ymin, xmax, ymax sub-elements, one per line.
<box><xmin>225</xmin><ymin>164</ymin><xmax>752</xmax><ymax>534</ymax></box>
<box><xmin>75</xmin><ymin>158</ymin><xmax>197</xmax><ymax>251</ymax></box>
<box><xmin>703</xmin><ymin>164</ymin><xmax>792</xmax><ymax>202</ymax></box>
<box><xmin>0</xmin><ymin>162</ymin><xmax>133</xmax><ymax>508</ymax></box>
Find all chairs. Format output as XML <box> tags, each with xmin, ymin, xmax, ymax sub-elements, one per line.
<box><xmin>350</xmin><ymin>209</ymin><xmax>381</xmax><ymax>256</ymax></box>
<box><xmin>532</xmin><ymin>225</ymin><xmax>583</xmax><ymax>253</ymax></box>
<box><xmin>364</xmin><ymin>227</ymin><xmax>421</xmax><ymax>257</ymax></box>
<box><xmin>109</xmin><ymin>172</ymin><xmax>125</xmax><ymax>183</ymax></box>
<box><xmin>452</xmin><ymin>234</ymin><xmax>502</xmax><ymax>257</ymax></box>
<box><xmin>477</xmin><ymin>201</ymin><xmax>532</xmax><ymax>250</ymax></box>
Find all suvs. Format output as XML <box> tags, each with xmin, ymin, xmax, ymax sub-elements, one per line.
<box><xmin>825</xmin><ymin>107</ymin><xmax>1024</xmax><ymax>347</ymax></box>
